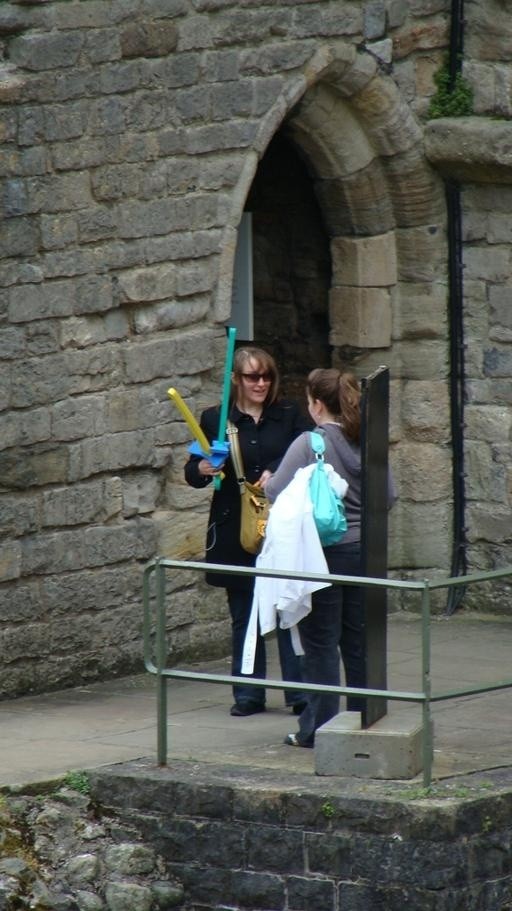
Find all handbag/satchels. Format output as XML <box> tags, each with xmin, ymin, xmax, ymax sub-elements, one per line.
<box><xmin>310</xmin><ymin>459</ymin><xmax>348</xmax><ymax>548</ymax></box>
<box><xmin>240</xmin><ymin>481</ymin><xmax>272</xmax><ymax>555</ymax></box>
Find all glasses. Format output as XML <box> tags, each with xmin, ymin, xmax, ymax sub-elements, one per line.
<box><xmin>240</xmin><ymin>373</ymin><xmax>273</xmax><ymax>382</ymax></box>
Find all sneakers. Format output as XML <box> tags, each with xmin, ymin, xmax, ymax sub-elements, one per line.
<box><xmin>288</xmin><ymin>733</ymin><xmax>300</xmax><ymax>745</ymax></box>
<box><xmin>294</xmin><ymin>700</ymin><xmax>307</xmax><ymax>715</ymax></box>
<box><xmin>231</xmin><ymin>701</ymin><xmax>266</xmax><ymax>716</ymax></box>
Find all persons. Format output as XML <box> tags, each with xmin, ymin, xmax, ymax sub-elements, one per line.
<box><xmin>184</xmin><ymin>346</ymin><xmax>318</xmax><ymax>715</ymax></box>
<box><xmin>260</xmin><ymin>369</ymin><xmax>397</xmax><ymax>748</ymax></box>
